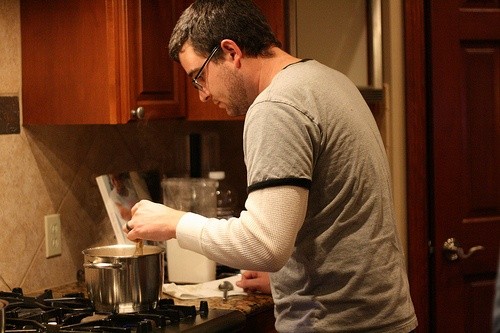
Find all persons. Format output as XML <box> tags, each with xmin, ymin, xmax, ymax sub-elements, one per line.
<box><xmin>121</xmin><ymin>0</ymin><xmax>418</xmax><ymax>333</ymax></box>
<box><xmin>109</xmin><ymin>172</ymin><xmax>138</xmax><ymax>222</ymax></box>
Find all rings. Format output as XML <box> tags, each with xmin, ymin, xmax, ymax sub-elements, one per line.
<box><xmin>125</xmin><ymin>221</ymin><xmax>132</xmax><ymax>231</ymax></box>
<box><xmin>125</xmin><ymin>222</ymin><xmax>132</xmax><ymax>231</ymax></box>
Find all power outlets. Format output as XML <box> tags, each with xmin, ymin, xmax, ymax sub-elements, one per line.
<box><xmin>45</xmin><ymin>215</ymin><xmax>62</xmax><ymax>258</ymax></box>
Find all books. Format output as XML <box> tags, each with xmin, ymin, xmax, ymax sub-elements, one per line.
<box><xmin>96</xmin><ymin>171</ymin><xmax>167</xmax><ymax>250</ymax></box>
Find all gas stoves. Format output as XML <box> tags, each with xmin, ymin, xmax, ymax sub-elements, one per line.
<box><xmin>0</xmin><ymin>288</ymin><xmax>243</xmax><ymax>333</ymax></box>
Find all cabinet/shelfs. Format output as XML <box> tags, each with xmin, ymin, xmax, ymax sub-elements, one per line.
<box><xmin>22</xmin><ymin>0</ymin><xmax>290</xmax><ymax>126</ymax></box>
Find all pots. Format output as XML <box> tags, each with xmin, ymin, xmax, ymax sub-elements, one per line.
<box><xmin>82</xmin><ymin>244</ymin><xmax>165</xmax><ymax>308</ymax></box>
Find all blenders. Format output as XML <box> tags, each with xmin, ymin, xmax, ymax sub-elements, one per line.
<box><xmin>163</xmin><ymin>178</ymin><xmax>218</xmax><ymax>284</ymax></box>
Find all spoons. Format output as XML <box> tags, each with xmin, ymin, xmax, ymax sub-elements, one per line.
<box><xmin>219</xmin><ymin>282</ymin><xmax>233</xmax><ymax>301</ymax></box>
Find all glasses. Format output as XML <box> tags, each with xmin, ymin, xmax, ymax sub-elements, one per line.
<box><xmin>191</xmin><ymin>43</ymin><xmax>220</xmax><ymax>91</ymax></box>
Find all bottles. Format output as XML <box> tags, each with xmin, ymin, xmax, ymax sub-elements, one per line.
<box><xmin>208</xmin><ymin>170</ymin><xmax>233</xmax><ymax>219</ymax></box>
